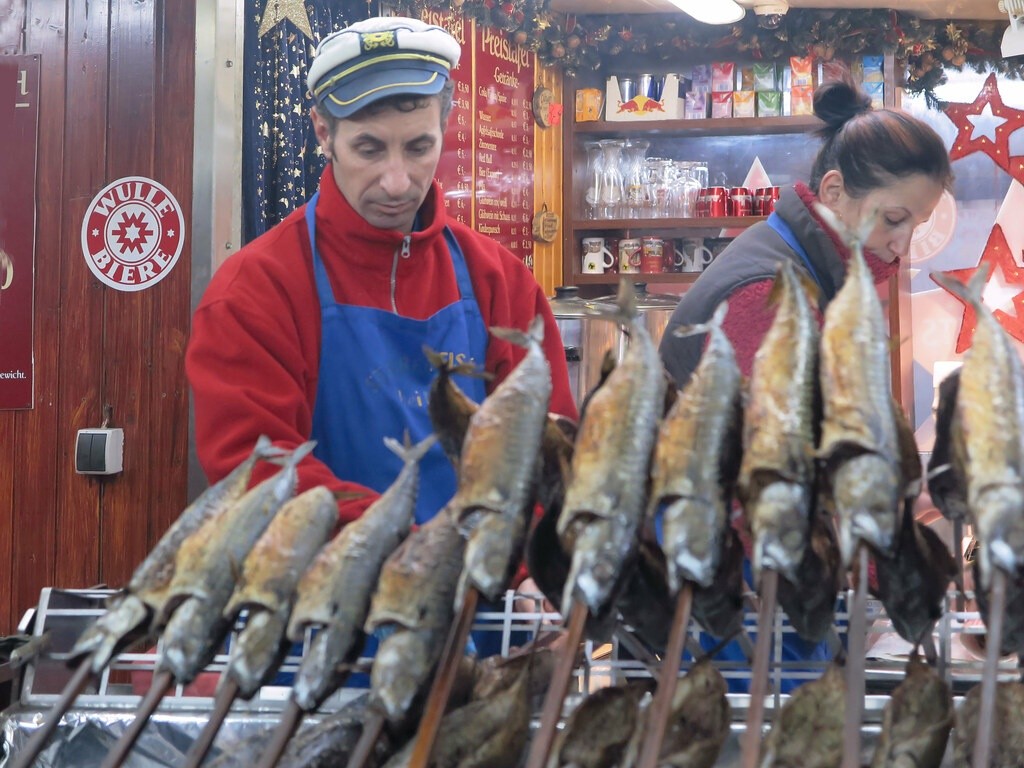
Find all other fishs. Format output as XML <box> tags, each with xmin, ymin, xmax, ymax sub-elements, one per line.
<box><xmin>68</xmin><ymin>246</ymin><xmax>1023</xmax><ymax>768</ymax></box>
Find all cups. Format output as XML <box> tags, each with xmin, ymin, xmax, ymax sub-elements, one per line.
<box><xmin>580</xmin><ymin>138</ymin><xmax>780</xmax><ymax>218</ymax></box>
<box><xmin>581</xmin><ymin>236</ymin><xmax>712</xmax><ymax>275</ymax></box>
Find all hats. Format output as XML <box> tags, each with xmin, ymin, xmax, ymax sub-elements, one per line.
<box><xmin>305</xmin><ymin>16</ymin><xmax>462</xmax><ymax>119</ymax></box>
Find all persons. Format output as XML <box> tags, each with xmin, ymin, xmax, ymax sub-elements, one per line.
<box><xmin>652</xmin><ymin>80</ymin><xmax>950</xmax><ymax>695</ymax></box>
<box><xmin>186</xmin><ymin>14</ymin><xmax>579</xmax><ymax>687</ymax></box>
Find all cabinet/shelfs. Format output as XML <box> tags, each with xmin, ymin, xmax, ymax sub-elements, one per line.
<box><xmin>562</xmin><ymin>14</ymin><xmax>914</xmax><ymax>431</ymax></box>
